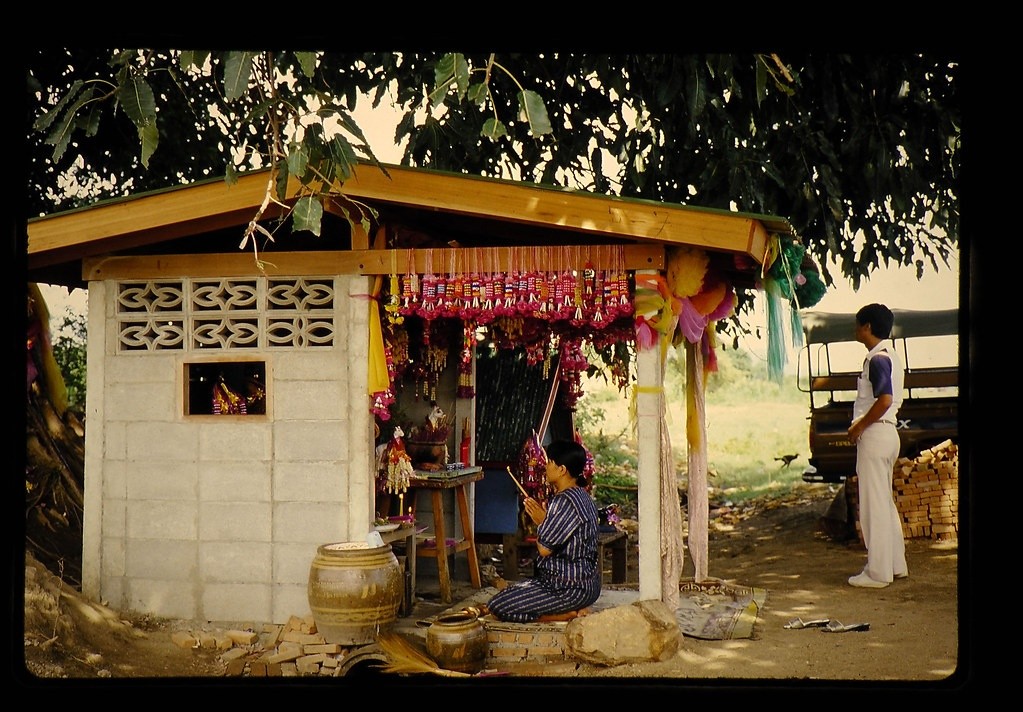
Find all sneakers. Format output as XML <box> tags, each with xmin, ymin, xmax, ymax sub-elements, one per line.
<box><xmin>893</xmin><ymin>573</ymin><xmax>909</xmax><ymax>577</ymax></box>
<box><xmin>848</xmin><ymin>571</ymin><xmax>889</xmax><ymax>588</ymax></box>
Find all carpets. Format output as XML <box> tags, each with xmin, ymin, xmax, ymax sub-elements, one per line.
<box><xmin>416</xmin><ymin>578</ymin><xmax>767</xmax><ymax>640</ymax></box>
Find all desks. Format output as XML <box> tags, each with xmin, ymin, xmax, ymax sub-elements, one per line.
<box><xmin>377</xmin><ymin>470</ymin><xmax>484</xmax><ymax>605</ymax></box>
<box><xmin>503</xmin><ymin>531</ymin><xmax>629</xmax><ymax>583</ymax></box>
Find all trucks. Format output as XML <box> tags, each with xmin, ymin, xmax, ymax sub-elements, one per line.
<box><xmin>797</xmin><ymin>309</ymin><xmax>959</xmax><ymax>482</ymax></box>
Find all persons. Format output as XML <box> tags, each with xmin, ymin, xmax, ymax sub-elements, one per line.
<box><xmin>848</xmin><ymin>303</ymin><xmax>909</xmax><ymax>588</ymax></box>
<box><xmin>486</xmin><ymin>441</ymin><xmax>601</xmax><ymax>622</ymax></box>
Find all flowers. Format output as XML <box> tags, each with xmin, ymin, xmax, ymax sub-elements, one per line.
<box><xmin>668</xmin><ymin>247</ymin><xmax>739</xmax><ymax>322</ymax></box>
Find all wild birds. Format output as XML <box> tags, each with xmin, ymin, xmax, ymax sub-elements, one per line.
<box><xmin>774</xmin><ymin>454</ymin><xmax>800</xmax><ymax>468</ymax></box>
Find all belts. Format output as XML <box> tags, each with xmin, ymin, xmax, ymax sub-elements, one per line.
<box><xmin>873</xmin><ymin>419</ymin><xmax>892</xmax><ymax>423</ymax></box>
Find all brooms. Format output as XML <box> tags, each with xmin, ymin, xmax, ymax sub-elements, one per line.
<box><xmin>367</xmin><ymin>628</ymin><xmax>475</xmax><ymax>678</ymax></box>
